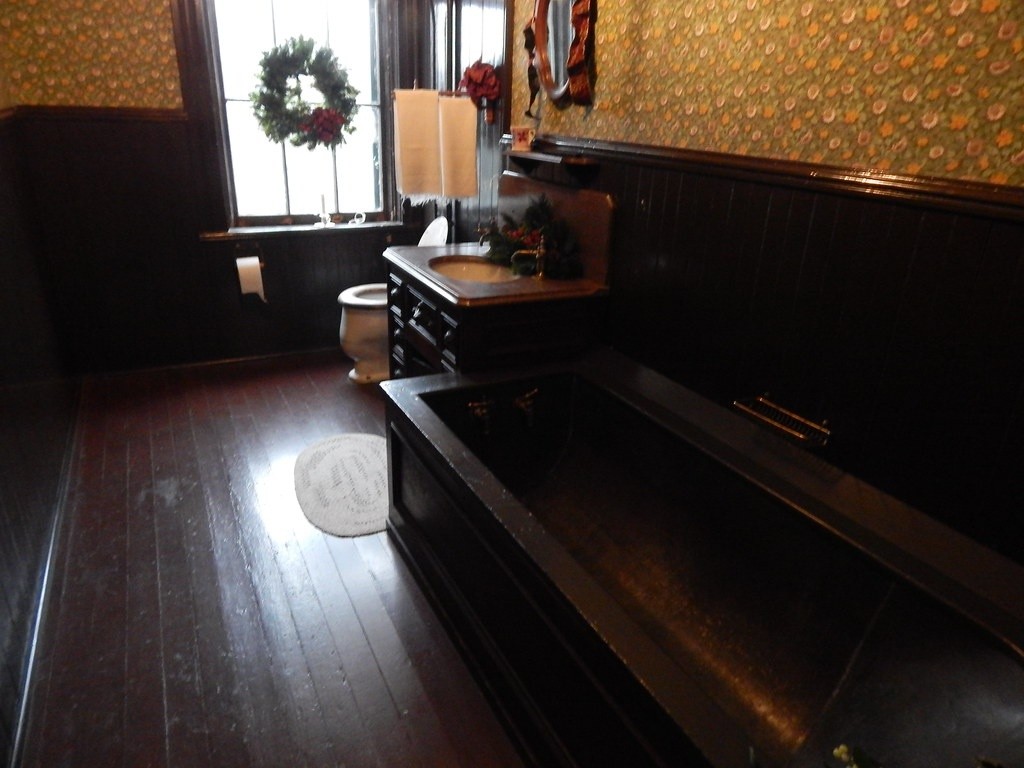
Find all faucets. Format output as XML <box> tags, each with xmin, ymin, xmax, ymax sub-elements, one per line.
<box><xmin>479</xmin><ymin>218</ymin><xmax>504</xmax><ymax>258</ymax></box>
<box><xmin>511</xmin><ymin>224</ymin><xmax>548</xmax><ymax>280</ymax></box>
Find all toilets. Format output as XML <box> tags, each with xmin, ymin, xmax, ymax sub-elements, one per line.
<box><xmin>336</xmin><ymin>215</ymin><xmax>449</xmax><ymax>384</ymax></box>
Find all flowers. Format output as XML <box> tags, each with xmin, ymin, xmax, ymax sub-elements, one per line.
<box><xmin>247</xmin><ymin>40</ymin><xmax>362</xmax><ymax>149</ymax></box>
<box><xmin>483</xmin><ymin>201</ymin><xmax>579</xmax><ymax>285</ymax></box>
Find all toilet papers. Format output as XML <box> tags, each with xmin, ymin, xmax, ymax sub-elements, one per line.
<box><xmin>236</xmin><ymin>255</ymin><xmax>267</xmax><ymax>304</ymax></box>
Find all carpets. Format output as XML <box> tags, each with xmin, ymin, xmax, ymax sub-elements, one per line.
<box><xmin>296</xmin><ymin>434</ymin><xmax>389</xmax><ymax>539</ymax></box>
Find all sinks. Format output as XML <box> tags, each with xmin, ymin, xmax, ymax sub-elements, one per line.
<box><xmin>428</xmin><ymin>255</ymin><xmax>522</xmax><ymax>284</ymax></box>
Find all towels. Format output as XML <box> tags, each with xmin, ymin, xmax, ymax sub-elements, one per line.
<box><xmin>390</xmin><ymin>88</ymin><xmax>480</xmax><ymax>205</ymax></box>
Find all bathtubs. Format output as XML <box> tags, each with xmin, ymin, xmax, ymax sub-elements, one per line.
<box><xmin>378</xmin><ymin>351</ymin><xmax>1024</xmax><ymax>768</ymax></box>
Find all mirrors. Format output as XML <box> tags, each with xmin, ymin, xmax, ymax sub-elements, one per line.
<box><xmin>530</xmin><ymin>1</ymin><xmax>578</xmax><ymax>109</ymax></box>
